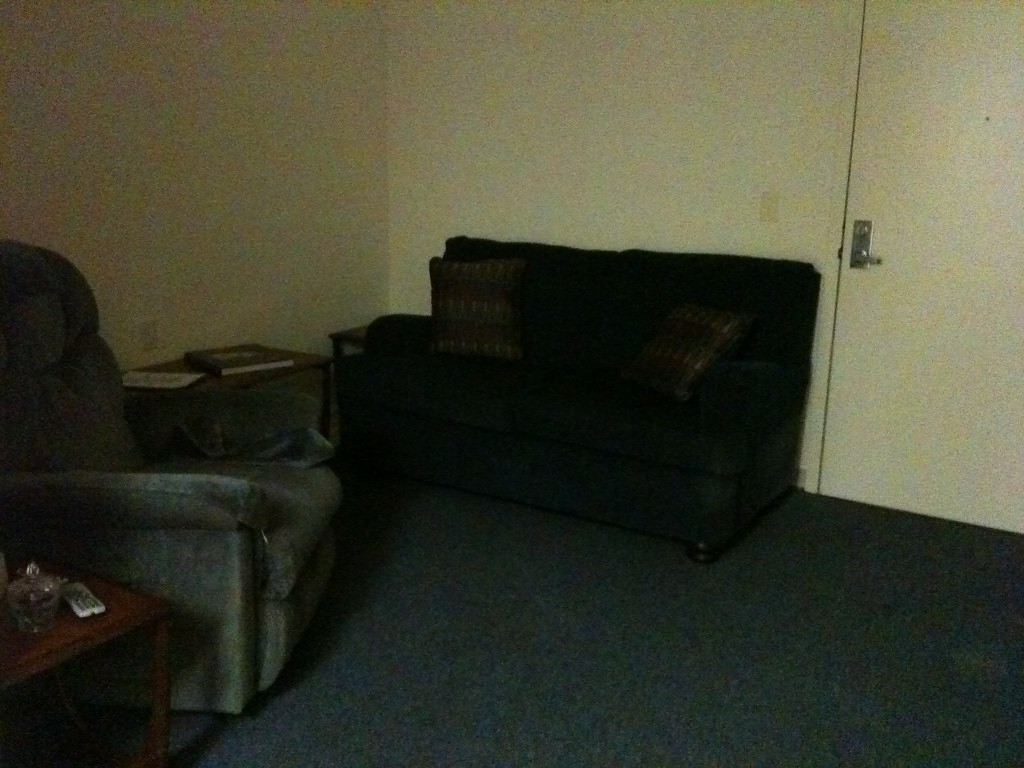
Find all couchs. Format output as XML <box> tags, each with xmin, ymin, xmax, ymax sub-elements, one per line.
<box><xmin>0</xmin><ymin>238</ymin><xmax>342</xmax><ymax>719</ymax></box>
<box><xmin>325</xmin><ymin>233</ymin><xmax>824</xmax><ymax>561</ymax></box>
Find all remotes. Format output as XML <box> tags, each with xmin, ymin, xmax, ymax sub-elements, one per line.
<box><xmin>61</xmin><ymin>581</ymin><xmax>106</xmax><ymax>619</ymax></box>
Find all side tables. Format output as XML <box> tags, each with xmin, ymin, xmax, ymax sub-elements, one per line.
<box><xmin>125</xmin><ymin>342</ymin><xmax>344</xmax><ymax>481</ymax></box>
<box><xmin>0</xmin><ymin>567</ymin><xmax>172</xmax><ymax>767</ymax></box>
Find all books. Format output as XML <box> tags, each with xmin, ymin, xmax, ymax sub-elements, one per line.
<box><xmin>184</xmin><ymin>343</ymin><xmax>294</xmax><ymax>377</ymax></box>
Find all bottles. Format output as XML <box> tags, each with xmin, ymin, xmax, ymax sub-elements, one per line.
<box><xmin>6</xmin><ymin>559</ymin><xmax>61</xmax><ymax>632</ymax></box>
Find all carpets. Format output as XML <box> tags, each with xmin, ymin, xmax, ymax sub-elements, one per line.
<box><xmin>177</xmin><ymin>484</ymin><xmax>1022</xmax><ymax>768</ymax></box>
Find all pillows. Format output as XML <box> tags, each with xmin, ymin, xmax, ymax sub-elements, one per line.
<box><xmin>425</xmin><ymin>256</ymin><xmax>536</xmax><ymax>363</ymax></box>
<box><xmin>624</xmin><ymin>295</ymin><xmax>747</xmax><ymax>393</ymax></box>
